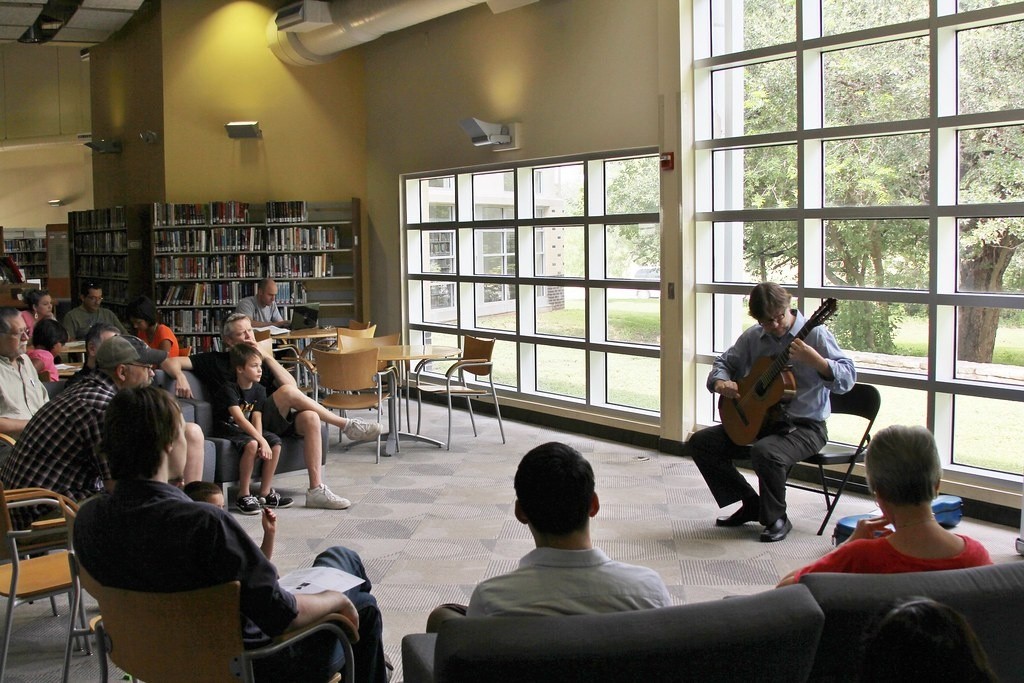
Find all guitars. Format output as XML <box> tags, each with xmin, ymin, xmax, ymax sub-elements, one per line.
<box><xmin>718</xmin><ymin>297</ymin><xmax>838</xmax><ymax>446</ymax></box>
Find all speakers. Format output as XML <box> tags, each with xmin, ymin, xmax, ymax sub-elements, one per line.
<box><xmin>226</xmin><ymin>121</ymin><xmax>259</xmax><ymax>138</ymax></box>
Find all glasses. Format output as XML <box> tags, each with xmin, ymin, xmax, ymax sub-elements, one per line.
<box><xmin>126</xmin><ymin>362</ymin><xmax>154</xmax><ymax>373</ymax></box>
<box><xmin>757</xmin><ymin>303</ymin><xmax>788</xmax><ymax>326</ymax></box>
<box><xmin>85</xmin><ymin>295</ymin><xmax>103</xmax><ymax>303</ymax></box>
<box><xmin>0</xmin><ymin>329</ymin><xmax>30</xmax><ymax>340</ymax></box>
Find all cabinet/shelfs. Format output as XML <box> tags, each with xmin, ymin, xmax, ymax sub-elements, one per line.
<box><xmin>4</xmin><ymin>251</ymin><xmax>48</xmax><ymax>278</ymax></box>
<box><xmin>65</xmin><ymin>196</ymin><xmax>371</xmax><ymax>357</ymax></box>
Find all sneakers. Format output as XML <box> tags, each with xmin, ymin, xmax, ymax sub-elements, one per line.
<box><xmin>344</xmin><ymin>418</ymin><xmax>383</xmax><ymax>441</ymax></box>
<box><xmin>304</xmin><ymin>487</ymin><xmax>352</xmax><ymax>507</ymax></box>
<box><xmin>234</xmin><ymin>494</ymin><xmax>262</xmax><ymax>515</ymax></box>
<box><xmin>257</xmin><ymin>488</ymin><xmax>293</xmax><ymax>508</ymax></box>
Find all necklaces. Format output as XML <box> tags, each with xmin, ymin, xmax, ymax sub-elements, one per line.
<box><xmin>895</xmin><ymin>517</ymin><xmax>935</xmax><ymax>525</ymax></box>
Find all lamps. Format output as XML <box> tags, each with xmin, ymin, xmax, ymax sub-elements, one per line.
<box><xmin>458</xmin><ymin>118</ymin><xmax>510</xmax><ymax>148</ymax></box>
<box><xmin>48</xmin><ymin>200</ymin><xmax>64</xmax><ymax>207</ymax></box>
<box><xmin>224</xmin><ymin>121</ymin><xmax>263</xmax><ymax>139</ymax></box>
<box><xmin>84</xmin><ymin>142</ymin><xmax>120</xmax><ymax>154</ymax></box>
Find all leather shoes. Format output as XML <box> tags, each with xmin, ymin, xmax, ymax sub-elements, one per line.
<box><xmin>760</xmin><ymin>511</ymin><xmax>793</xmax><ymax>542</ymax></box>
<box><xmin>717</xmin><ymin>497</ymin><xmax>763</xmax><ymax>525</ymax></box>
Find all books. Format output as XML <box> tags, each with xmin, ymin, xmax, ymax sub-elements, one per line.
<box><xmin>72</xmin><ymin>205</ymin><xmax>129</xmax><ymax>322</ymax></box>
<box><xmin>152</xmin><ymin>253</ymin><xmax>334</xmax><ymax>361</ymax></box>
<box><xmin>154</xmin><ymin>199</ymin><xmax>305</xmax><ymax>226</ymax></box>
<box><xmin>5</xmin><ymin>238</ymin><xmax>48</xmax><ymax>290</ymax></box>
<box><xmin>155</xmin><ymin>225</ymin><xmax>340</xmax><ymax>252</ymax></box>
<box><xmin>0</xmin><ymin>255</ymin><xmax>24</xmax><ymax>283</ymax></box>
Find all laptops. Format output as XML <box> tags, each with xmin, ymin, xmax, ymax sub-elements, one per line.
<box><xmin>280</xmin><ymin>302</ymin><xmax>320</xmax><ymax>330</ymax></box>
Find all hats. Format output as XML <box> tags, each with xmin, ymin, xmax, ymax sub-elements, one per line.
<box><xmin>94</xmin><ymin>334</ymin><xmax>167</xmax><ymax>367</ymax></box>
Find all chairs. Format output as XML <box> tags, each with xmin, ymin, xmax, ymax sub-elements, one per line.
<box><xmin>254</xmin><ymin>317</ymin><xmax>505</xmax><ymax>453</ymax></box>
<box><xmin>39</xmin><ymin>379</ymin><xmax>215</xmax><ymax>485</ymax></box>
<box><xmin>153</xmin><ymin>370</ymin><xmax>330</xmax><ymax>511</ymax></box>
<box><xmin>0</xmin><ymin>479</ymin><xmax>95</xmax><ymax>683</ymax></box>
<box><xmin>89</xmin><ymin>584</ymin><xmax>361</xmax><ymax>683</ymax></box>
<box><xmin>401</xmin><ymin>583</ymin><xmax>825</xmax><ymax>683</ymax></box>
<box><xmin>784</xmin><ymin>382</ymin><xmax>881</xmax><ymax>536</ymax></box>
<box><xmin>722</xmin><ymin>559</ymin><xmax>1024</xmax><ymax>683</ymax></box>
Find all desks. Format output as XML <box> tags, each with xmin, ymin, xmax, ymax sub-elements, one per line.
<box><xmin>54</xmin><ymin>362</ymin><xmax>84</xmax><ymax>380</ymax></box>
<box><xmin>345</xmin><ymin>346</ymin><xmax>461</xmax><ymax>448</ymax></box>
<box><xmin>57</xmin><ymin>338</ymin><xmax>87</xmax><ymax>363</ymax></box>
<box><xmin>268</xmin><ymin>328</ymin><xmax>337</xmax><ymax>355</ymax></box>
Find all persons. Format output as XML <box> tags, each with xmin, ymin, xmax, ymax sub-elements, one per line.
<box><xmin>73</xmin><ymin>385</ymin><xmax>386</xmax><ymax>683</ymax></box>
<box><xmin>58</xmin><ymin>280</ymin><xmax>132</xmax><ymax>361</ymax></box>
<box><xmin>0</xmin><ymin>307</ymin><xmax>50</xmax><ymax>440</ymax></box>
<box><xmin>65</xmin><ymin>323</ymin><xmax>120</xmax><ymax>387</ymax></box>
<box><xmin>179</xmin><ymin>482</ymin><xmax>276</xmax><ymax>564</ymax></box>
<box><xmin>776</xmin><ymin>425</ymin><xmax>994</xmax><ymax>586</ymax></box>
<box><xmin>2</xmin><ymin>334</ymin><xmax>168</xmax><ymax>547</ymax></box>
<box><xmin>126</xmin><ymin>296</ymin><xmax>180</xmax><ymax>370</ymax></box>
<box><xmin>26</xmin><ymin>319</ymin><xmax>68</xmax><ymax>383</ymax></box>
<box><xmin>19</xmin><ymin>291</ymin><xmax>59</xmax><ymax>337</ymax></box>
<box><xmin>686</xmin><ymin>282</ymin><xmax>858</xmax><ymax>544</ymax></box>
<box><xmin>159</xmin><ymin>314</ymin><xmax>383</xmax><ymax>509</ymax></box>
<box><xmin>236</xmin><ymin>279</ymin><xmax>291</xmax><ymax>328</ymax></box>
<box><xmin>213</xmin><ymin>342</ymin><xmax>294</xmax><ymax>516</ymax></box>
<box><xmin>425</xmin><ymin>441</ymin><xmax>674</xmax><ymax>633</ymax></box>
